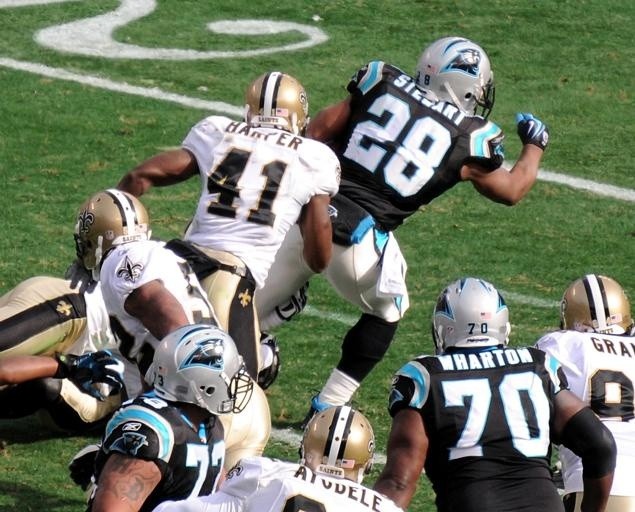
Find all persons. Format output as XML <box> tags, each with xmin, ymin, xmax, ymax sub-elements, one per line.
<box><xmin>118</xmin><ymin>69</ymin><xmax>343</xmax><ymax>386</ymax></box>
<box><xmin>0</xmin><ymin>276</ymin><xmax>127</xmax><ymax>434</ymax></box>
<box><xmin>261</xmin><ymin>34</ymin><xmax>549</xmax><ymax>432</ymax></box>
<box><xmin>88</xmin><ymin>325</ymin><xmax>255</xmax><ymax>512</ymax></box>
<box><xmin>74</xmin><ymin>188</ymin><xmax>272</xmax><ymax>489</ymax></box>
<box><xmin>152</xmin><ymin>404</ymin><xmax>401</xmax><ymax>511</ymax></box>
<box><xmin>530</xmin><ymin>274</ymin><xmax>634</xmax><ymax>512</ymax></box>
<box><xmin>371</xmin><ymin>276</ymin><xmax>617</xmax><ymax>512</ymax></box>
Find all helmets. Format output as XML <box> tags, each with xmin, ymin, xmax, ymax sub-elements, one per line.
<box><xmin>243</xmin><ymin>70</ymin><xmax>311</xmax><ymax>136</ymax></box>
<box><xmin>431</xmin><ymin>276</ymin><xmax>511</xmax><ymax>356</ymax></box>
<box><xmin>297</xmin><ymin>405</ymin><xmax>377</xmax><ymax>486</ymax></box>
<box><xmin>152</xmin><ymin>322</ymin><xmax>246</xmax><ymax>416</ymax></box>
<box><xmin>75</xmin><ymin>187</ymin><xmax>153</xmax><ymax>270</ymax></box>
<box><xmin>414</xmin><ymin>35</ymin><xmax>494</xmax><ymax>115</ymax></box>
<box><xmin>559</xmin><ymin>272</ymin><xmax>634</xmax><ymax>337</ymax></box>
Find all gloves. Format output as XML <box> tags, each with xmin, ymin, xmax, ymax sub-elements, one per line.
<box><xmin>515</xmin><ymin>110</ymin><xmax>550</xmax><ymax>152</ymax></box>
<box><xmin>52</xmin><ymin>348</ymin><xmax>126</xmax><ymax>403</ymax></box>
<box><xmin>64</xmin><ymin>258</ymin><xmax>99</xmax><ymax>296</ymax></box>
